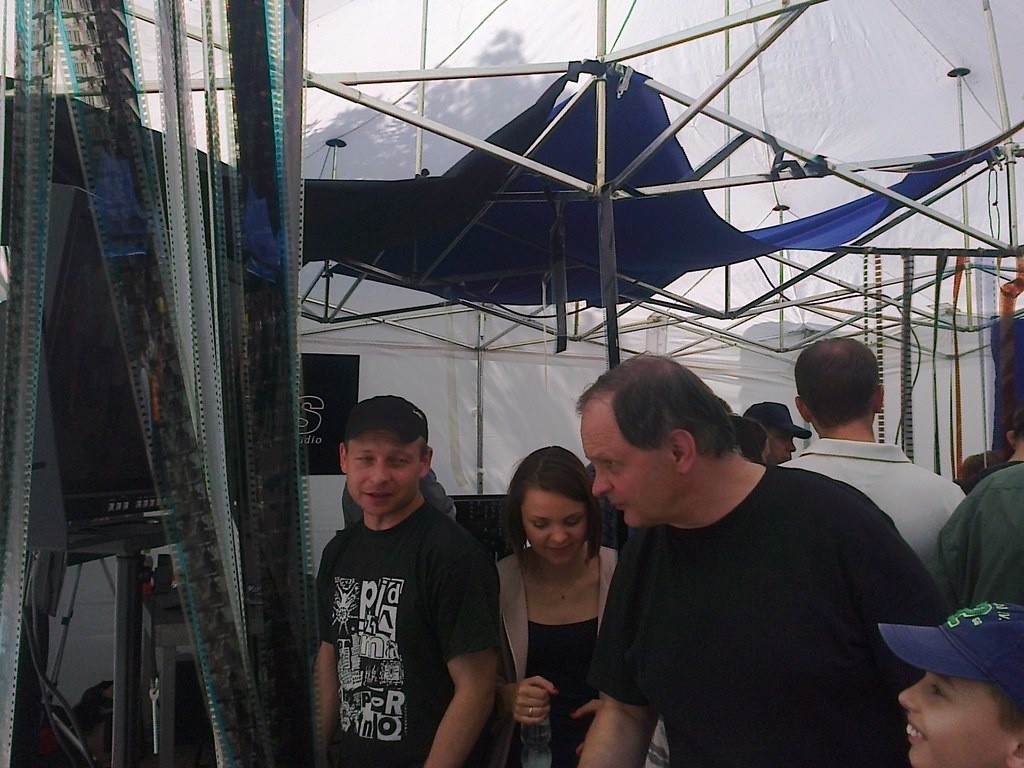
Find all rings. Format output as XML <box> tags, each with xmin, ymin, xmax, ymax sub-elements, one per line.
<box><xmin>528</xmin><ymin>707</ymin><xmax>533</xmax><ymax>716</ymax></box>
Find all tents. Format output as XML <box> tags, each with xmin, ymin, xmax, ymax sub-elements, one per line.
<box><xmin>47</xmin><ymin>61</ymin><xmax>1024</xmax><ymax>705</ymax></box>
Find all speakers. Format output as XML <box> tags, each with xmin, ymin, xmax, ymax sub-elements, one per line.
<box><xmin>41</xmin><ymin>184</ymin><xmax>173</xmax><ymax>530</ymax></box>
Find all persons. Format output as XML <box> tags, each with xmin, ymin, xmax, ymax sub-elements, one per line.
<box><xmin>778</xmin><ymin>339</ymin><xmax>967</xmax><ymax>569</ymax></box>
<box><xmin>342</xmin><ymin>466</ymin><xmax>457</xmax><ymax>529</ymax></box>
<box><xmin>577</xmin><ymin>356</ymin><xmax>953</xmax><ymax>768</ymax></box>
<box><xmin>715</xmin><ymin>395</ymin><xmax>812</xmax><ymax>466</ymax></box>
<box><xmin>313</xmin><ymin>395</ymin><xmax>501</xmax><ymax>768</ymax></box>
<box><xmin>878</xmin><ymin>603</ymin><xmax>1024</xmax><ymax>768</ymax></box>
<box><xmin>928</xmin><ymin>406</ymin><xmax>1024</xmax><ymax>610</ymax></box>
<box><xmin>486</xmin><ymin>446</ymin><xmax>670</xmax><ymax>768</ymax></box>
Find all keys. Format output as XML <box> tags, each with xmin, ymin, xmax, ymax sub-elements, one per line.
<box><xmin>149</xmin><ymin>688</ymin><xmax>159</xmax><ymax>754</ymax></box>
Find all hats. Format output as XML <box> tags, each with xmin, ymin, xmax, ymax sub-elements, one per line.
<box><xmin>743</xmin><ymin>402</ymin><xmax>812</xmax><ymax>439</ymax></box>
<box><xmin>345</xmin><ymin>395</ymin><xmax>429</xmax><ymax>443</ymax></box>
<box><xmin>878</xmin><ymin>602</ymin><xmax>1024</xmax><ymax>710</ymax></box>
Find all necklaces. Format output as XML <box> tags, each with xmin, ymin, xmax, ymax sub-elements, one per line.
<box><xmin>535</xmin><ymin>559</ymin><xmax>585</xmax><ymax>599</ymax></box>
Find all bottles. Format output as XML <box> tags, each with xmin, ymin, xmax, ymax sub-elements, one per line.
<box><xmin>519</xmin><ymin>684</ymin><xmax>553</xmax><ymax>767</ymax></box>
